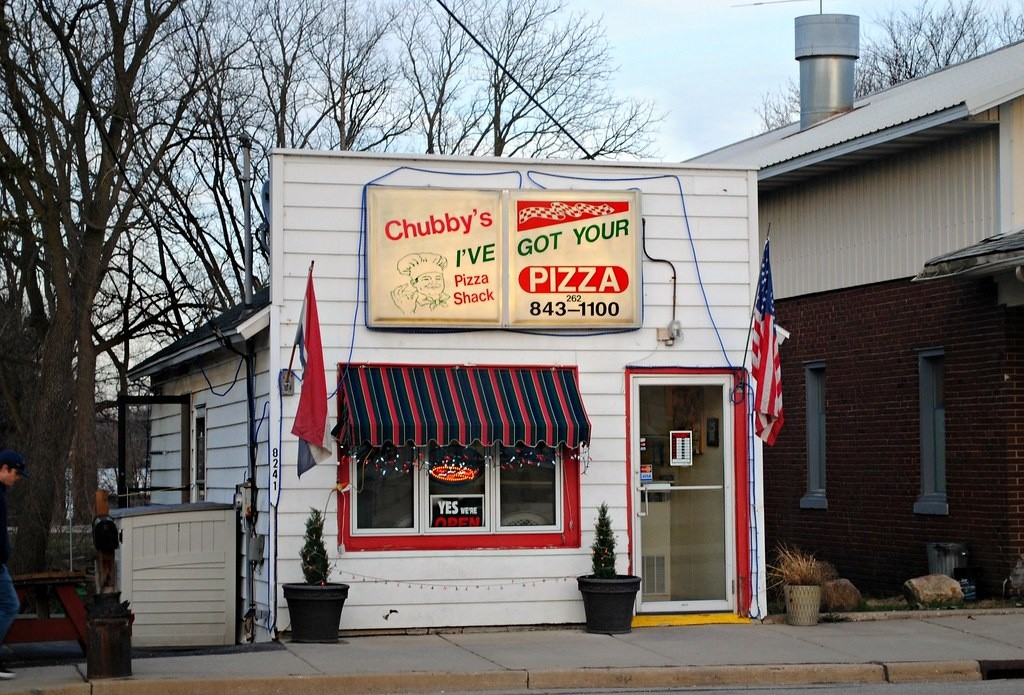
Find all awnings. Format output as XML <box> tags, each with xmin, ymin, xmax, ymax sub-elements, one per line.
<box><xmin>336</xmin><ymin>361</ymin><xmax>593</xmax><ymax>453</ymax></box>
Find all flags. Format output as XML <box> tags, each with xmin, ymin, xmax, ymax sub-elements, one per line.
<box><xmin>753</xmin><ymin>239</ymin><xmax>782</xmax><ymax>445</ymax></box>
<box><xmin>287</xmin><ymin>280</ymin><xmax>332</xmax><ymax>481</ymax></box>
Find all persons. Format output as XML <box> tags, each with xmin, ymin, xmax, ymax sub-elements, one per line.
<box><xmin>0</xmin><ymin>448</ymin><xmax>28</xmax><ymax>680</ymax></box>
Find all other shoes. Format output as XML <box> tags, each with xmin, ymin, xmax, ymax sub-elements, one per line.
<box><xmin>0</xmin><ymin>663</ymin><xmax>16</xmax><ymax>678</ymax></box>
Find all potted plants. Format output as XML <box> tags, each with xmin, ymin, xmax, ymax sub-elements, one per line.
<box><xmin>283</xmin><ymin>508</ymin><xmax>349</xmax><ymax>645</ymax></box>
<box><xmin>577</xmin><ymin>505</ymin><xmax>642</xmax><ymax>633</ymax></box>
<box><xmin>767</xmin><ymin>543</ymin><xmax>825</xmax><ymax>627</ymax></box>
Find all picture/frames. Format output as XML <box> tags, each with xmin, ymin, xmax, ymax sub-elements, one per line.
<box><xmin>706</xmin><ymin>418</ymin><xmax>719</xmax><ymax>447</ymax></box>
<box><xmin>672</xmin><ymin>386</ymin><xmax>704</xmax><ymax>454</ymax></box>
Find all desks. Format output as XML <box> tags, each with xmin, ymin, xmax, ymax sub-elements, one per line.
<box><xmin>4</xmin><ymin>576</ymin><xmax>91</xmax><ymax>657</ymax></box>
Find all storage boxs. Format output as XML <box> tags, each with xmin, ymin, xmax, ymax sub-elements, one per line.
<box><xmin>641</xmin><ymin>464</ymin><xmax>653</xmax><ymax>482</ymax></box>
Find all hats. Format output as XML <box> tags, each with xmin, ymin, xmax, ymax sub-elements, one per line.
<box><xmin>0</xmin><ymin>449</ymin><xmax>31</xmax><ymax>477</ymax></box>
